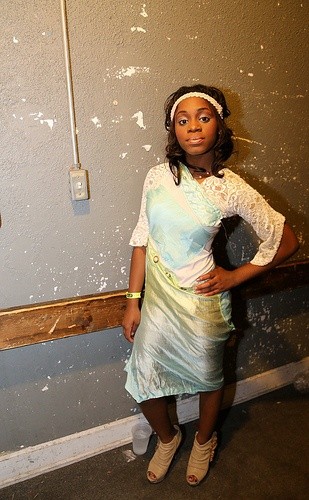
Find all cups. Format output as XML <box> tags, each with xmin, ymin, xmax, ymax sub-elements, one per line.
<box><xmin>131</xmin><ymin>422</ymin><xmax>152</xmax><ymax>455</ymax></box>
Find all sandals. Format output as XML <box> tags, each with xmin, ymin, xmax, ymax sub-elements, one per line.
<box><xmin>186</xmin><ymin>431</ymin><xmax>217</xmax><ymax>485</ymax></box>
<box><xmin>147</xmin><ymin>425</ymin><xmax>182</xmax><ymax>483</ymax></box>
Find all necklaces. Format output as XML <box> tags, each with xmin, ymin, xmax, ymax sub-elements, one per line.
<box><xmin>190</xmin><ymin>170</ymin><xmax>211</xmax><ymax>180</ymax></box>
<box><xmin>122</xmin><ymin>84</ymin><xmax>300</xmax><ymax>486</ymax></box>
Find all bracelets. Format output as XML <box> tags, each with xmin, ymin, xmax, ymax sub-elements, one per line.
<box><xmin>126</xmin><ymin>292</ymin><xmax>142</xmax><ymax>299</ymax></box>
